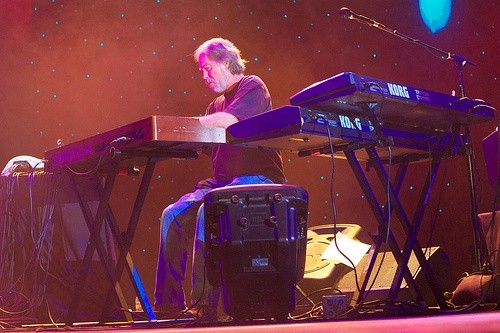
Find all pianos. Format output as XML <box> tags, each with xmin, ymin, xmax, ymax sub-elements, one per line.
<box><xmin>43</xmin><ymin>115</ymin><xmax>227</xmax><ymax>327</ymax></box>
<box><xmin>226</xmin><ymin>71</ymin><xmax>496</xmax><ymax>316</ymax></box>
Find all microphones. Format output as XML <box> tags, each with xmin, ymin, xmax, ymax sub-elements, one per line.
<box><xmin>339</xmin><ymin>8</ymin><xmax>383</xmax><ymax>28</ymax></box>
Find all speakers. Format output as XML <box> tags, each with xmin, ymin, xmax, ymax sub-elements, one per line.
<box><xmin>294</xmin><ymin>223</ymin><xmax>369</xmax><ymax>317</ymax></box>
<box><xmin>333</xmin><ymin>246</ymin><xmax>457</xmax><ymax>312</ymax></box>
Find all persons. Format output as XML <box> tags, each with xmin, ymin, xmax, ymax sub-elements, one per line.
<box><xmin>135</xmin><ymin>37</ymin><xmax>286</xmax><ymax>320</ymax></box>
